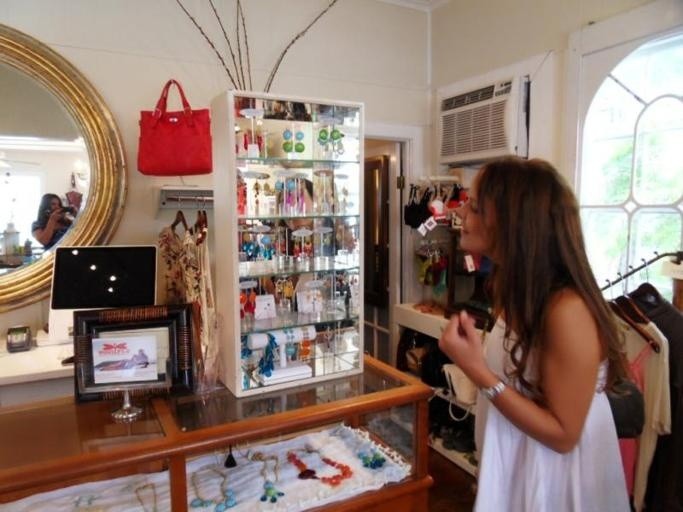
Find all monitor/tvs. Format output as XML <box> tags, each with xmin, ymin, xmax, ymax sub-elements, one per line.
<box><xmin>51</xmin><ymin>244</ymin><xmax>155</xmax><ymax>366</ymax></box>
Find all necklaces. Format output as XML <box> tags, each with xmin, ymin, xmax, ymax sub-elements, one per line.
<box><xmin>287</xmin><ymin>444</ymin><xmax>353</xmax><ymax>487</ymax></box>
<box><xmin>332</xmin><ymin>428</ymin><xmax>386</xmax><ymax>469</ymax></box>
<box><xmin>251</xmin><ymin>452</ymin><xmax>285</xmax><ymax>503</ymax></box>
<box><xmin>191</xmin><ymin>464</ymin><xmax>237</xmax><ymax>512</ymax></box>
<box><xmin>135</xmin><ymin>482</ymin><xmax>159</xmax><ymax>512</ymax></box>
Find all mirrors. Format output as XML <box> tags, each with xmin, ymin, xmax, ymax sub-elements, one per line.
<box><xmin>0</xmin><ymin>22</ymin><xmax>129</xmax><ymax>315</ymax></box>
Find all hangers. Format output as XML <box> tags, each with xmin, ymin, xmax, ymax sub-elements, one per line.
<box><xmin>171</xmin><ymin>193</ymin><xmax>210</xmax><ymax>247</ymax></box>
<box><xmin>605</xmin><ymin>259</ymin><xmax>661</xmax><ymax>355</ymax></box>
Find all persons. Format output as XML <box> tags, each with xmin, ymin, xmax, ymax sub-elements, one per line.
<box><xmin>266</xmin><ymin>176</ymin><xmax>356</xmax><ymax>344</ymax></box>
<box><xmin>32</xmin><ymin>193</ymin><xmax>78</xmax><ymax>250</ymax></box>
<box><xmin>439</xmin><ymin>155</ymin><xmax>632</xmax><ymax>512</ymax></box>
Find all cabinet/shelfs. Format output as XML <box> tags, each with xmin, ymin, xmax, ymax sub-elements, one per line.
<box><xmin>1</xmin><ymin>348</ymin><xmax>435</xmax><ymax>512</ymax></box>
<box><xmin>208</xmin><ymin>88</ymin><xmax>367</xmax><ymax>399</ymax></box>
<box><xmin>392</xmin><ymin>303</ymin><xmax>493</xmax><ymax>471</ymax></box>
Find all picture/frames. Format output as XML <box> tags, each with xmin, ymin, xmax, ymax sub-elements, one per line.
<box><xmin>73</xmin><ymin>302</ymin><xmax>198</xmax><ymax>405</ymax></box>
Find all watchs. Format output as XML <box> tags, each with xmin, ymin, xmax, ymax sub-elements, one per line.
<box><xmin>479</xmin><ymin>382</ymin><xmax>509</xmax><ymax>400</ymax></box>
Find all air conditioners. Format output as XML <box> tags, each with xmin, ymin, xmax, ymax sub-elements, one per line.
<box><xmin>435</xmin><ymin>76</ymin><xmax>528</xmax><ymax>166</ymax></box>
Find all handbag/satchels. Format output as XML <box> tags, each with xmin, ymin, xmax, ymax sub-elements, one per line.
<box><xmin>442</xmin><ymin>364</ymin><xmax>477</xmax><ymax>420</ymax></box>
<box><xmin>605</xmin><ymin>356</ymin><xmax>645</xmax><ymax>437</ymax></box>
<box><xmin>137</xmin><ymin>80</ymin><xmax>212</xmax><ymax>176</ymax></box>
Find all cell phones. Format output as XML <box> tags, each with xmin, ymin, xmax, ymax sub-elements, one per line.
<box><xmin>59</xmin><ymin>205</ymin><xmax>75</xmax><ymax>214</ymax></box>
